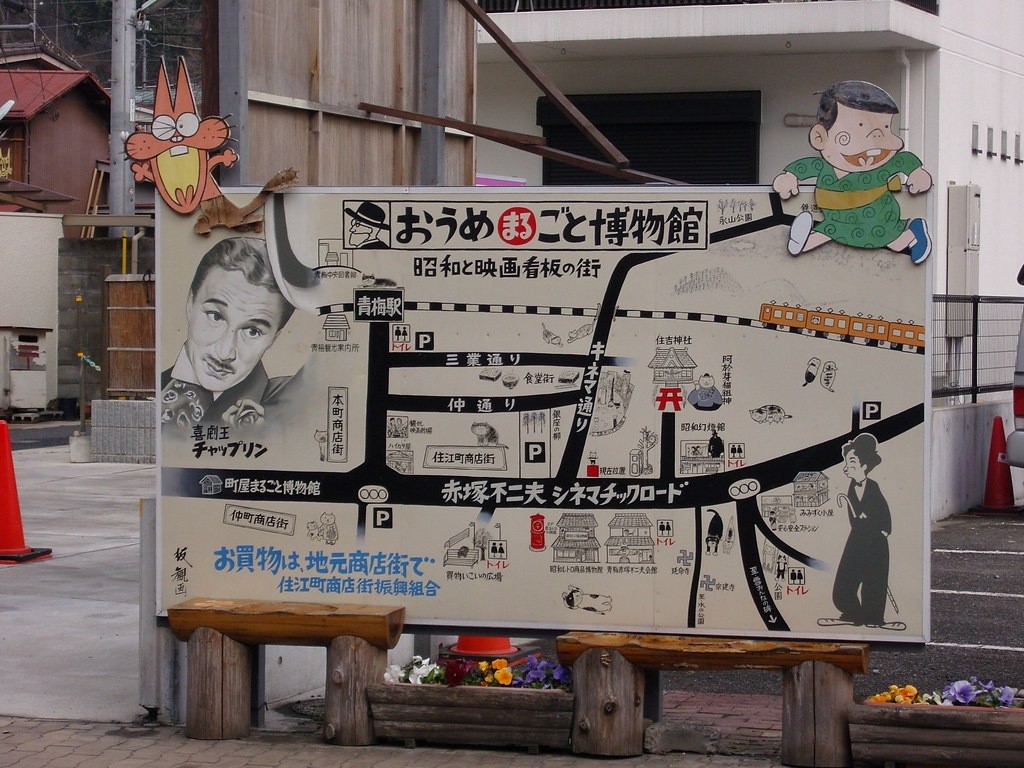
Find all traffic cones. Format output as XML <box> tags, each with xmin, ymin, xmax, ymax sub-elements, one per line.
<box><xmin>0</xmin><ymin>419</ymin><xmax>53</xmax><ymax>564</ymax></box>
<box><xmin>967</xmin><ymin>416</ymin><xmax>1024</xmax><ymax>515</ymax></box>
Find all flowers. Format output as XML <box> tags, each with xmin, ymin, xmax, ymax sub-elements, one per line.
<box><xmin>385</xmin><ymin>651</ymin><xmax>568</xmax><ymax>688</ymax></box>
<box><xmin>866</xmin><ymin>671</ymin><xmax>1024</xmax><ymax>706</ymax></box>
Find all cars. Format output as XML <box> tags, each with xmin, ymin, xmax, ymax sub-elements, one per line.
<box><xmin>1004</xmin><ymin>263</ymin><xmax>1024</xmax><ymax>468</ymax></box>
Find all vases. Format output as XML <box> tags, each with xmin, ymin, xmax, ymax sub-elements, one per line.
<box><xmin>848</xmin><ymin>702</ymin><xmax>1024</xmax><ymax>768</ymax></box>
<box><xmin>366</xmin><ymin>685</ymin><xmax>575</xmax><ymax>754</ymax></box>
